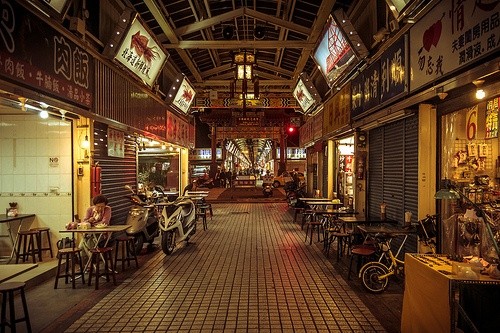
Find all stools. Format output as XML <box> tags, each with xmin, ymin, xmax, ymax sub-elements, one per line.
<box><xmin>292</xmin><ymin>207</ymin><xmax>376</xmax><ymax>281</ymax></box>
<box><xmin>194</xmin><ymin>203</ymin><xmax>212</xmax><ymax>231</ymax></box>
<box><xmin>0</xmin><ymin>226</ymin><xmax>139</xmax><ymax>333</ymax></box>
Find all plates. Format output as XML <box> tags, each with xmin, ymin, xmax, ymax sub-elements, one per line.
<box><xmin>95</xmin><ymin>226</ymin><xmax>107</xmax><ymax>228</ymax></box>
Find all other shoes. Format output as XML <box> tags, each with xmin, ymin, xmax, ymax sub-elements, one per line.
<box><xmin>85</xmin><ymin>267</ymin><xmax>90</xmax><ymax>273</ymax></box>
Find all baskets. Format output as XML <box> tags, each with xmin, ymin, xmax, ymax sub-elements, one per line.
<box><xmin>415</xmin><ymin>219</ymin><xmax>436</xmax><ymax>239</ymax></box>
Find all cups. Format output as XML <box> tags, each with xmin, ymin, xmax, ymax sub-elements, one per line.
<box><xmin>381</xmin><ymin>213</ymin><xmax>386</xmax><ymax>221</ymax></box>
<box><xmin>405</xmin><ymin>222</ymin><xmax>411</xmax><ymax>232</ymax></box>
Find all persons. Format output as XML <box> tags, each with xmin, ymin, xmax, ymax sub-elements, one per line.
<box><xmin>262</xmin><ymin>170</ymin><xmax>272</xmax><ymax>188</ymax></box>
<box><xmin>215</xmin><ymin>169</ymin><xmax>232</xmax><ymax>188</ymax></box>
<box><xmin>290</xmin><ymin>172</ymin><xmax>298</xmax><ymax>190</ymax></box>
<box><xmin>233</xmin><ymin>168</ymin><xmax>252</xmax><ymax>179</ymax></box>
<box><xmin>198</xmin><ymin>169</ymin><xmax>209</xmax><ymax>186</ymax></box>
<box><xmin>75</xmin><ymin>194</ymin><xmax>111</xmax><ymax>272</ymax></box>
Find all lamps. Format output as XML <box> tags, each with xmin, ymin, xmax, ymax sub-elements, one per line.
<box><xmin>82</xmin><ymin>117</ymin><xmax>89</xmax><ymax>148</ymax></box>
<box><xmin>434</xmin><ymin>178</ymin><xmax>500</xmax><ymax>275</ymax></box>
<box><xmin>472</xmin><ymin>80</ymin><xmax>485</xmax><ymax>99</ymax></box>
<box><xmin>39</xmin><ymin>104</ymin><xmax>49</xmax><ymax>118</ymax></box>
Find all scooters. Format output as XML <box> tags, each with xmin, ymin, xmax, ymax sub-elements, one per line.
<box><xmin>262</xmin><ymin>184</ymin><xmax>273</xmax><ymax>197</ymax></box>
<box><xmin>124</xmin><ymin>185</ymin><xmax>159</xmax><ymax>255</ymax></box>
<box><xmin>192</xmin><ymin>178</ymin><xmax>214</xmax><ymax>189</ymax></box>
<box><xmin>283</xmin><ymin>182</ymin><xmax>302</xmax><ymax>208</ymax></box>
<box><xmin>154</xmin><ymin>183</ymin><xmax>197</xmax><ymax>255</ymax></box>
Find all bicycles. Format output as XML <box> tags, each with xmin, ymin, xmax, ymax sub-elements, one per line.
<box><xmin>359</xmin><ymin>212</ymin><xmax>438</xmax><ymax>292</ymax></box>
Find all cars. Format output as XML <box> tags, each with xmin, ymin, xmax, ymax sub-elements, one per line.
<box><xmin>273</xmin><ymin>170</ymin><xmax>306</xmax><ymax>187</ymax></box>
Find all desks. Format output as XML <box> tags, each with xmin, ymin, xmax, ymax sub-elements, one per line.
<box><xmin>189</xmin><ymin>190</ymin><xmax>210</xmax><ymax>202</ymax></box>
<box><xmin>299</xmin><ymin>197</ymin><xmax>416</xmax><ymax>248</ymax></box>
<box><xmin>0</xmin><ymin>213</ymin><xmax>35</xmax><ymax>264</ymax></box>
<box><xmin>400</xmin><ymin>252</ymin><xmax>500</xmax><ymax>333</ymax></box>
<box><xmin>0</xmin><ymin>263</ymin><xmax>38</xmax><ymax>284</ymax></box>
<box><xmin>59</xmin><ymin>224</ymin><xmax>132</xmax><ymax>282</ymax></box>
